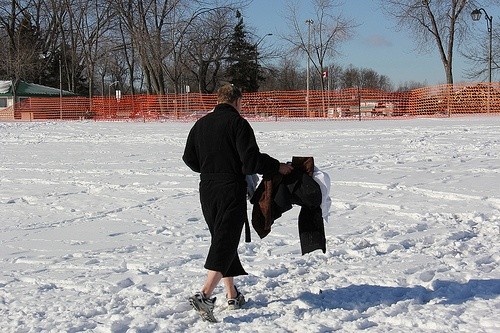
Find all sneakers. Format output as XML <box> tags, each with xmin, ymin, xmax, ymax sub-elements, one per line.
<box><xmin>188</xmin><ymin>291</ymin><xmax>218</xmax><ymax>323</ymax></box>
<box><xmin>226</xmin><ymin>285</ymin><xmax>245</xmax><ymax>310</ymax></box>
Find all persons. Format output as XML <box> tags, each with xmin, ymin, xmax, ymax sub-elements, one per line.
<box><xmin>182</xmin><ymin>84</ymin><xmax>293</xmax><ymax>322</ymax></box>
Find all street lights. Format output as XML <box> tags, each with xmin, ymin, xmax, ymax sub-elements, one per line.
<box><xmin>471</xmin><ymin>8</ymin><xmax>492</xmax><ymax>114</ymax></box>
<box><xmin>305</xmin><ymin>19</ymin><xmax>316</xmax><ymax>118</ymax></box>
